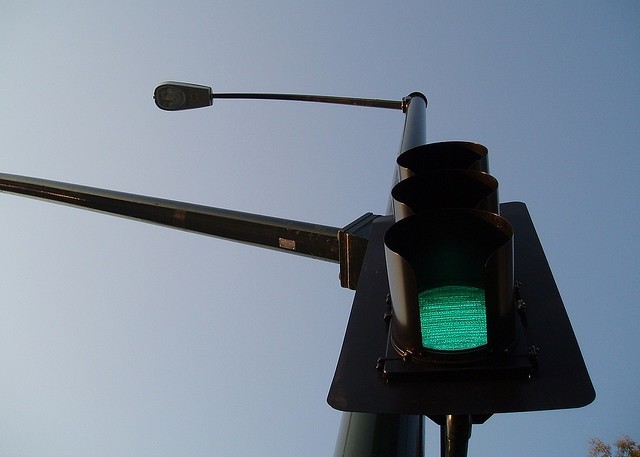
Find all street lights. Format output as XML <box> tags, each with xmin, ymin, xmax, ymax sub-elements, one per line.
<box><xmin>153</xmin><ymin>80</ymin><xmax>427</xmax><ymax>456</ymax></box>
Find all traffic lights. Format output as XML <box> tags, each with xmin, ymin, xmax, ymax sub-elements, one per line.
<box><xmin>326</xmin><ymin>140</ymin><xmax>596</xmax><ymax>414</ymax></box>
